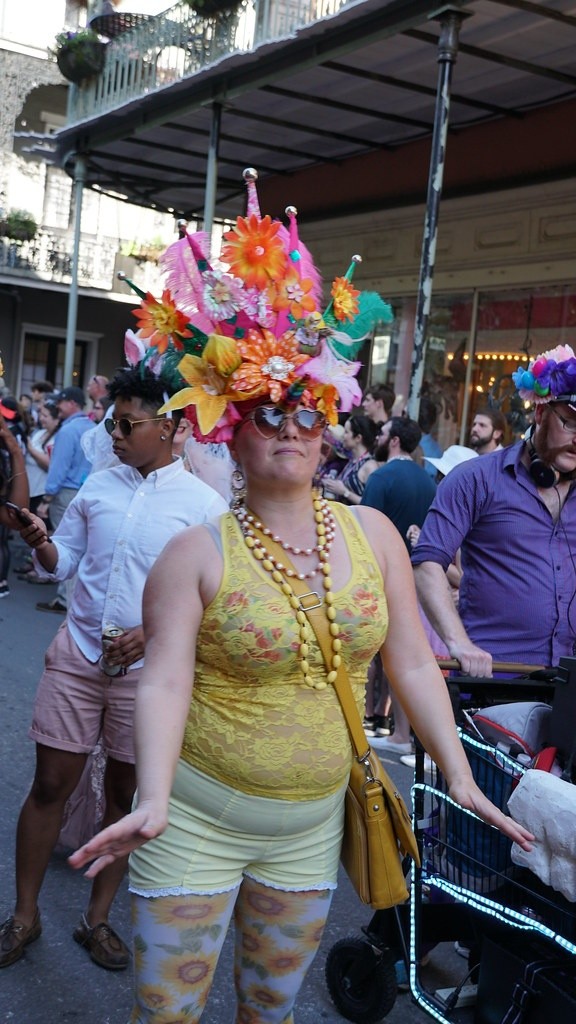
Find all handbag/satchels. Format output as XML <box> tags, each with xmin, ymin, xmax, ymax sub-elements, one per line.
<box><xmin>335</xmin><ymin>751</ymin><xmax>423</xmax><ymax>908</ymax></box>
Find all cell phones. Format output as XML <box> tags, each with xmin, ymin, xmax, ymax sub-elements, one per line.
<box><xmin>6</xmin><ymin>502</ymin><xmax>52</xmax><ymax>543</ymax></box>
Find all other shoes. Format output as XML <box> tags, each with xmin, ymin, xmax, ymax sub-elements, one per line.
<box><xmin>36</xmin><ymin>600</ymin><xmax>66</xmax><ymax>615</ymax></box>
<box><xmin>27</xmin><ymin>576</ymin><xmax>54</xmax><ymax>584</ymax></box>
<box><xmin>395</xmin><ymin>961</ymin><xmax>411</xmax><ymax>989</ymax></box>
<box><xmin>454</xmin><ymin>940</ymin><xmax>470</xmax><ymax>960</ymax></box>
<box><xmin>0</xmin><ymin>580</ymin><xmax>10</xmax><ymax>598</ymax></box>
<box><xmin>14</xmin><ymin>566</ymin><xmax>34</xmax><ymax>574</ymax></box>
<box><xmin>362</xmin><ymin>713</ymin><xmax>390</xmax><ymax>737</ymax></box>
<box><xmin>18</xmin><ymin>571</ymin><xmax>37</xmax><ymax>580</ymax></box>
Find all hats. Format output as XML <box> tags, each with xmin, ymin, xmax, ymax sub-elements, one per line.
<box><xmin>0</xmin><ymin>394</ymin><xmax>18</xmax><ymax>420</ymax></box>
<box><xmin>47</xmin><ymin>386</ymin><xmax>86</xmax><ymax>406</ymax></box>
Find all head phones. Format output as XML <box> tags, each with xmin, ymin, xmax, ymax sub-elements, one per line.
<box><xmin>524</xmin><ymin>424</ymin><xmax>576</xmax><ymax>488</ymax></box>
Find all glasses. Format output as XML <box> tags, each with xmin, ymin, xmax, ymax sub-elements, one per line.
<box><xmin>233</xmin><ymin>406</ymin><xmax>330</xmax><ymax>442</ymax></box>
<box><xmin>104</xmin><ymin>411</ymin><xmax>171</xmax><ymax>437</ymax></box>
<box><xmin>545</xmin><ymin>403</ymin><xmax>576</xmax><ymax>433</ymax></box>
<box><xmin>91</xmin><ymin>374</ymin><xmax>99</xmax><ymax>388</ymax></box>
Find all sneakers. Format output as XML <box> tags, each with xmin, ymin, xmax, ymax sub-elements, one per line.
<box><xmin>399</xmin><ymin>754</ymin><xmax>438</xmax><ymax>775</ymax></box>
<box><xmin>0</xmin><ymin>905</ymin><xmax>44</xmax><ymax>968</ymax></box>
<box><xmin>73</xmin><ymin>915</ymin><xmax>132</xmax><ymax>969</ymax></box>
<box><xmin>365</xmin><ymin>735</ymin><xmax>412</xmax><ymax>753</ymax></box>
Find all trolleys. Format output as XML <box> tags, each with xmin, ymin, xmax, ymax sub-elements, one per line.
<box><xmin>324</xmin><ymin>657</ymin><xmax>575</xmax><ymax>1024</ymax></box>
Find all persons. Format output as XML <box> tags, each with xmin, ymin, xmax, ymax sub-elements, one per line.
<box><xmin>1</xmin><ymin>364</ymin><xmax>232</xmax><ymax>968</ymax></box>
<box><xmin>0</xmin><ymin>370</ymin><xmax>123</xmax><ymax>616</ymax></box>
<box><xmin>172</xmin><ymin>414</ymin><xmax>235</xmax><ymax>504</ymax></box>
<box><xmin>316</xmin><ymin>380</ymin><xmax>510</xmax><ymax>774</ymax></box>
<box><xmin>410</xmin><ymin>342</ymin><xmax>576</xmax><ymax>959</ymax></box>
<box><xmin>66</xmin><ymin>387</ymin><xmax>535</xmax><ymax>1024</ymax></box>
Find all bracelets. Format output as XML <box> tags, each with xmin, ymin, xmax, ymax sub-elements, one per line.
<box><xmin>343</xmin><ymin>491</ymin><xmax>350</xmax><ymax>497</ymax></box>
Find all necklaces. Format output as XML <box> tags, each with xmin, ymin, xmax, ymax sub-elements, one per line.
<box><xmin>184</xmin><ymin>449</ymin><xmax>196</xmax><ymax>475</ymax></box>
<box><xmin>228</xmin><ymin>491</ymin><xmax>343</xmax><ymax>689</ymax></box>
<box><xmin>339</xmin><ymin>448</ymin><xmax>369</xmax><ymax>482</ymax></box>
